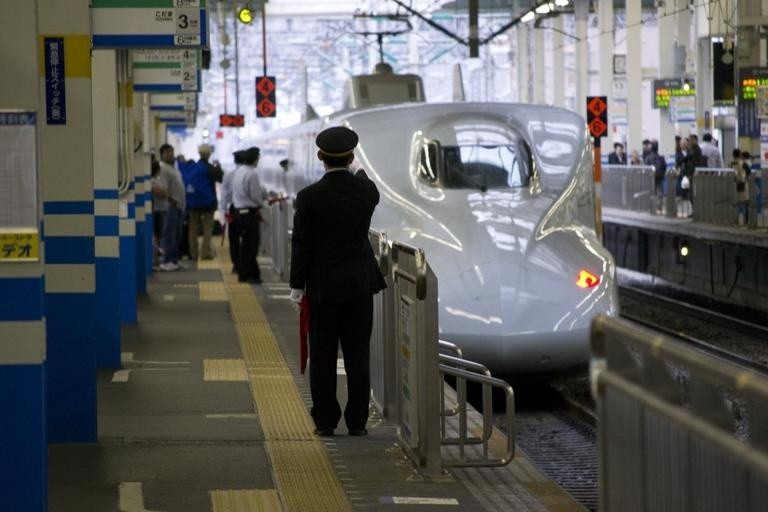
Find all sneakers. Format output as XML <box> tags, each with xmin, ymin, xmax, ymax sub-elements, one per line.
<box><xmin>159</xmin><ymin>262</ymin><xmax>179</xmax><ymax>270</ymax></box>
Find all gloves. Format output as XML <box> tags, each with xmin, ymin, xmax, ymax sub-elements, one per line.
<box><xmin>288</xmin><ymin>289</ymin><xmax>304</xmax><ymax>303</ymax></box>
<box><xmin>350</xmin><ymin>161</ymin><xmax>363</xmax><ymax>175</ymax></box>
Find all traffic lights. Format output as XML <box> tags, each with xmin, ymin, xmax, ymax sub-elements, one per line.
<box><xmin>238</xmin><ymin>9</ymin><xmax>254</xmax><ymax>22</ymax></box>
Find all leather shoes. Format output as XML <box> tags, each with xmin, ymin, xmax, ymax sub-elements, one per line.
<box><xmin>349</xmin><ymin>428</ymin><xmax>368</xmax><ymax>436</ymax></box>
<box><xmin>314</xmin><ymin>428</ymin><xmax>334</xmax><ymax>436</ymax></box>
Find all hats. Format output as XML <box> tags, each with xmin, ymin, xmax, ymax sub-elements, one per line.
<box><xmin>233</xmin><ymin>148</ymin><xmax>257</xmax><ymax>161</ymax></box>
<box><xmin>316</xmin><ymin>126</ymin><xmax>358</xmax><ymax>156</ymax></box>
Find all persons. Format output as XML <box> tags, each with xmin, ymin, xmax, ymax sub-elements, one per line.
<box><xmin>150</xmin><ymin>142</ymin><xmax>288</xmax><ymax>284</ymax></box>
<box><xmin>290</xmin><ymin>127</ymin><xmax>388</xmax><ymax>437</ymax></box>
<box><xmin>609</xmin><ymin>132</ymin><xmax>763</xmax><ymax>225</ymax></box>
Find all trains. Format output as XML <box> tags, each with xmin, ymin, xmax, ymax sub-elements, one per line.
<box><xmin>237</xmin><ymin>63</ymin><xmax>622</xmax><ymax>377</ymax></box>
